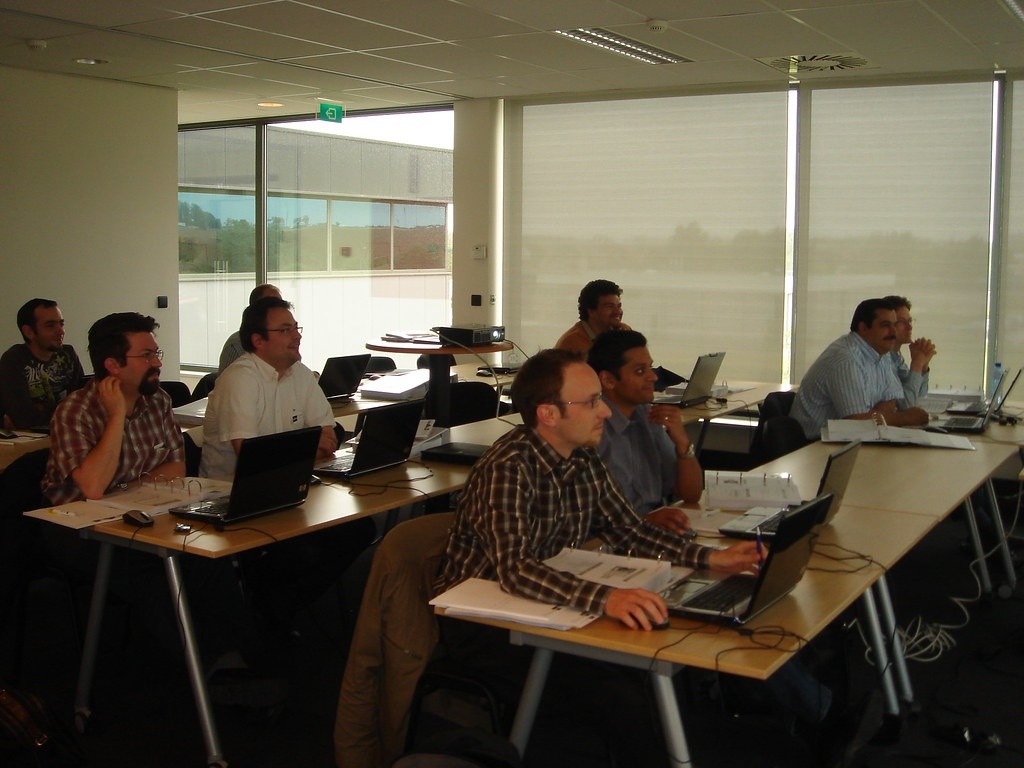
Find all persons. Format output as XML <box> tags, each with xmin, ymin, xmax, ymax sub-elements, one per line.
<box><xmin>38</xmin><ymin>312</ymin><xmax>187</xmax><ymax>690</ymax></box>
<box><xmin>196</xmin><ymin>296</ymin><xmax>377</xmax><ymax>669</ymax></box>
<box><xmin>588</xmin><ymin>329</ymin><xmax>886</xmax><ymax>768</ymax></box>
<box><xmin>786</xmin><ymin>299</ymin><xmax>930</xmax><ymax>443</ymax></box>
<box><xmin>552</xmin><ymin>279</ymin><xmax>632</xmax><ymax>363</ymax></box>
<box><xmin>218</xmin><ymin>285</ymin><xmax>284</xmax><ymax>374</ymax></box>
<box><xmin>433</xmin><ymin>348</ymin><xmax>771</xmax><ymax>768</ymax></box>
<box><xmin>880</xmin><ymin>295</ymin><xmax>1024</xmax><ymax>574</ymax></box>
<box><xmin>0</xmin><ymin>298</ymin><xmax>87</xmax><ymax>431</ymax></box>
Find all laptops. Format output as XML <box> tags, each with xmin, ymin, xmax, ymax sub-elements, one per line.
<box><xmin>650</xmin><ymin>351</ymin><xmax>726</xmax><ymax>407</ymax></box>
<box><xmin>312</xmin><ymin>397</ymin><xmax>426</xmax><ymax>479</ymax></box>
<box><xmin>169</xmin><ymin>425</ymin><xmax>324</xmax><ymax>524</ymax></box>
<box><xmin>654</xmin><ymin>435</ymin><xmax>863</xmax><ymax>625</ymax></box>
<box><xmin>318</xmin><ymin>354</ymin><xmax>371</xmax><ymax>400</ymax></box>
<box><xmin>13</xmin><ymin>375</ymin><xmax>95</xmax><ymax>434</ymax></box>
<box><xmin>477</xmin><ymin>363</ymin><xmax>523</xmax><ymax>373</ymax></box>
<box><xmin>919</xmin><ymin>367</ymin><xmax>1022</xmax><ymax>433</ymax></box>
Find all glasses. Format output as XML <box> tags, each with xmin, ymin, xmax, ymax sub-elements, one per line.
<box><xmin>266</xmin><ymin>327</ymin><xmax>303</xmax><ymax>335</ymax></box>
<box><xmin>122</xmin><ymin>350</ymin><xmax>164</xmax><ymax>361</ymax></box>
<box><xmin>549</xmin><ymin>392</ymin><xmax>605</xmax><ymax>409</ymax></box>
<box><xmin>896</xmin><ymin>318</ymin><xmax>915</xmax><ymax>327</ymax></box>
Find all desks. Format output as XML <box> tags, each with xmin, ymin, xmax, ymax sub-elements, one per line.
<box><xmin>365</xmin><ymin>333</ymin><xmax>514</xmax><ymax>356</ymax></box>
<box><xmin>0</xmin><ymin>363</ymin><xmax>1024</xmax><ymax>768</ymax></box>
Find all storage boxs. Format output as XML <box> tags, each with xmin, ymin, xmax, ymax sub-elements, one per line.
<box><xmin>362</xmin><ymin>368</ymin><xmax>431</xmax><ymax>401</ymax></box>
<box><xmin>450</xmin><ymin>372</ymin><xmax>459</xmax><ymax>384</ymax></box>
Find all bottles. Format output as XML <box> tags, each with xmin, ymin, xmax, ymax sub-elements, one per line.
<box><xmin>991</xmin><ymin>362</ymin><xmax>1005</xmax><ymax>404</ymax></box>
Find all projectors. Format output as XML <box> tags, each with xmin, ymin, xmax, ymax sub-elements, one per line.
<box><xmin>439</xmin><ymin>323</ymin><xmax>506</xmax><ymax>346</ymax></box>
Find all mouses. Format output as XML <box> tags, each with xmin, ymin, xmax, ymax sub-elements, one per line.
<box><xmin>123</xmin><ymin>510</ymin><xmax>154</xmax><ymax>527</ymax></box>
<box><xmin>977</xmin><ymin>412</ymin><xmax>1000</xmax><ymax>421</ymax></box>
<box><xmin>476</xmin><ymin>369</ymin><xmax>493</xmax><ymax>376</ymax></box>
<box><xmin>681</xmin><ymin>529</ymin><xmax>697</xmax><ymax>539</ymax></box>
<box><xmin>922</xmin><ymin>425</ymin><xmax>948</xmax><ymax>434</ymax></box>
<box><xmin>0</xmin><ymin>428</ymin><xmax>18</xmax><ymax>439</ymax></box>
<box><xmin>309</xmin><ymin>475</ymin><xmax>322</xmax><ymax>483</ymax></box>
<box><xmin>620</xmin><ymin>612</ymin><xmax>670</xmax><ymax>630</ymax></box>
